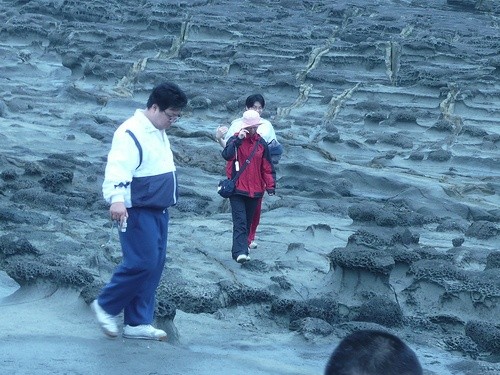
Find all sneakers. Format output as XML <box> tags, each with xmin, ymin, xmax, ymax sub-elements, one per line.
<box><xmin>122</xmin><ymin>324</ymin><xmax>167</xmax><ymax>340</ymax></box>
<box><xmin>90</xmin><ymin>300</ymin><xmax>119</xmax><ymax>339</ymax></box>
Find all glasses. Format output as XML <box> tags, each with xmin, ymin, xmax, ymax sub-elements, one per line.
<box><xmin>163</xmin><ymin>111</ymin><xmax>182</xmax><ymax>123</ymax></box>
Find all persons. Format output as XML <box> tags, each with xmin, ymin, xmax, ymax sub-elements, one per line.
<box><xmin>221</xmin><ymin>110</ymin><xmax>277</xmax><ymax>263</ymax></box>
<box><xmin>325</xmin><ymin>330</ymin><xmax>423</xmax><ymax>375</ymax></box>
<box><xmin>216</xmin><ymin>94</ymin><xmax>277</xmax><ymax>249</ymax></box>
<box><xmin>90</xmin><ymin>81</ymin><xmax>188</xmax><ymax>342</ymax></box>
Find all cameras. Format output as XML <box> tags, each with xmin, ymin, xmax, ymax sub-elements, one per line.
<box><xmin>118</xmin><ymin>215</ymin><xmax>128</xmax><ymax>232</ymax></box>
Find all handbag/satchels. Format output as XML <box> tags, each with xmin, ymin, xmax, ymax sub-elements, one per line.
<box><xmin>217</xmin><ymin>179</ymin><xmax>236</xmax><ymax>198</ymax></box>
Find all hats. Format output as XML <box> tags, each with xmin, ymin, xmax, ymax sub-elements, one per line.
<box><xmin>240</xmin><ymin>109</ymin><xmax>263</xmax><ymax>129</ymax></box>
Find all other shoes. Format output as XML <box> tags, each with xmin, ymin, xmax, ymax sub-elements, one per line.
<box><xmin>249</xmin><ymin>242</ymin><xmax>257</xmax><ymax>249</ymax></box>
<box><xmin>237</xmin><ymin>255</ymin><xmax>247</xmax><ymax>263</ymax></box>
<box><xmin>247</xmin><ymin>256</ymin><xmax>250</xmax><ymax>261</ymax></box>
